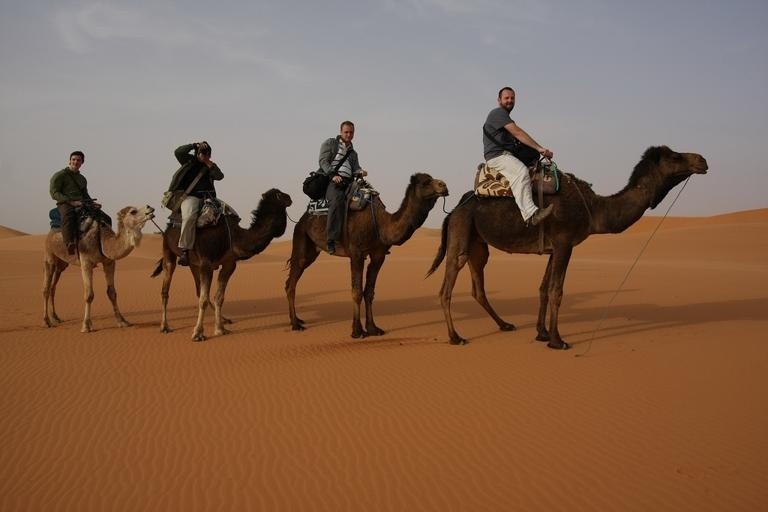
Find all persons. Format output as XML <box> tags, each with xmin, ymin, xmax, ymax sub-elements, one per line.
<box><xmin>49</xmin><ymin>143</ymin><xmax>100</xmax><ymax>255</ymax></box>
<box><xmin>482</xmin><ymin>87</ymin><xmax>555</xmax><ymax>228</ymax></box>
<box><xmin>166</xmin><ymin>141</ymin><xmax>224</xmax><ymax>267</ymax></box>
<box><xmin>317</xmin><ymin>120</ymin><xmax>368</xmax><ymax>255</ymax></box>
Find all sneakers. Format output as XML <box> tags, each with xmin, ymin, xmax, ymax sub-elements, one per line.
<box><xmin>326</xmin><ymin>239</ymin><xmax>335</xmax><ymax>255</ymax></box>
<box><xmin>68</xmin><ymin>242</ymin><xmax>75</xmax><ymax>255</ymax></box>
<box><xmin>532</xmin><ymin>204</ymin><xmax>553</xmax><ymax>226</ymax></box>
<box><xmin>178</xmin><ymin>255</ymin><xmax>189</xmax><ymax>266</ymax></box>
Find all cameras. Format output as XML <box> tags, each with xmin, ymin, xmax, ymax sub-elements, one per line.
<box><xmin>198</xmin><ymin>143</ymin><xmax>210</xmax><ymax>155</ymax></box>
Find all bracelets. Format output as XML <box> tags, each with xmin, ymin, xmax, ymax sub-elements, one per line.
<box><xmin>193</xmin><ymin>143</ymin><xmax>198</xmax><ymax>150</ymax></box>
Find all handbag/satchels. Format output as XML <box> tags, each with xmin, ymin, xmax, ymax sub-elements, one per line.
<box><xmin>161</xmin><ymin>190</ymin><xmax>187</xmax><ymax>211</ymax></box>
<box><xmin>512</xmin><ymin>137</ymin><xmax>540</xmax><ymax>168</ymax></box>
<box><xmin>303</xmin><ymin>172</ymin><xmax>330</xmax><ymax>202</ymax></box>
